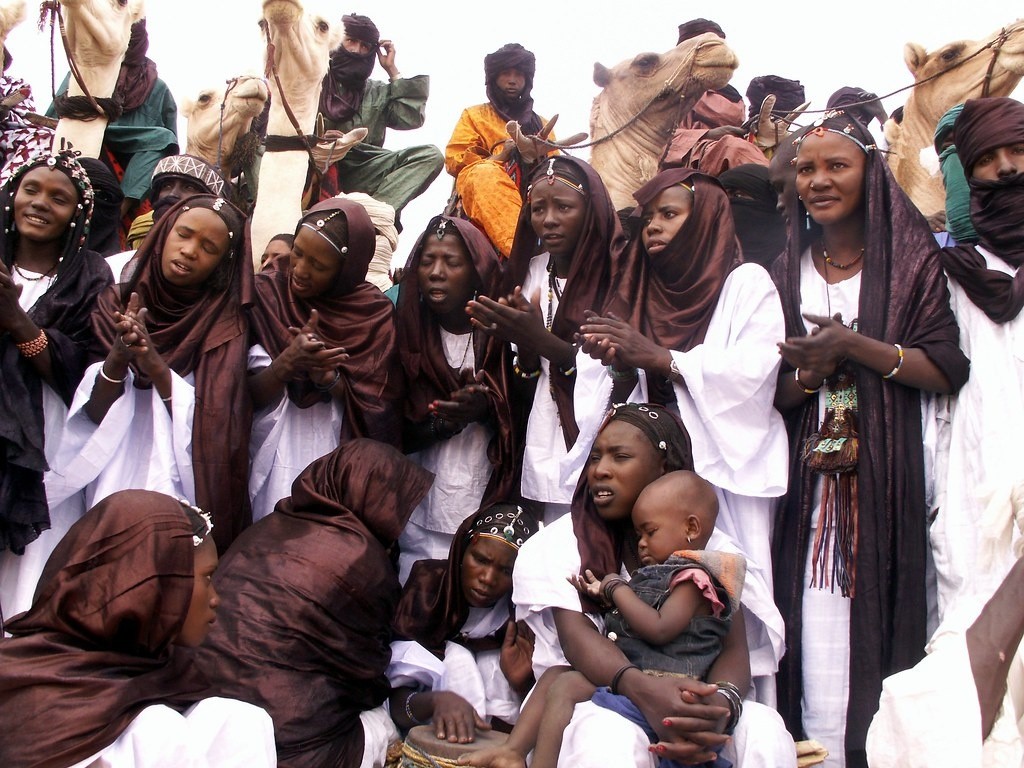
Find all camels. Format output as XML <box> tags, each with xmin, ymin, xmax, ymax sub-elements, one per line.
<box><xmin>180</xmin><ymin>75</ymin><xmax>268</xmax><ymax>175</ymax></box>
<box><xmin>885</xmin><ymin>20</ymin><xmax>1024</xmax><ymax>236</ymax></box>
<box><xmin>49</xmin><ymin>0</ymin><xmax>146</xmax><ymax>157</ymax></box>
<box><xmin>250</xmin><ymin>0</ymin><xmax>346</xmax><ymax>278</ymax></box>
<box><xmin>589</xmin><ymin>32</ymin><xmax>739</xmax><ymax>214</ymax></box>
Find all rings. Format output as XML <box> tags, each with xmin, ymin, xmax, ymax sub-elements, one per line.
<box><xmin>120</xmin><ymin>336</ymin><xmax>131</xmax><ymax>347</ymax></box>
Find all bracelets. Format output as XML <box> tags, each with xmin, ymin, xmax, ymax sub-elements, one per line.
<box><xmin>315</xmin><ymin>367</ymin><xmax>340</xmax><ymax>392</ymax></box>
<box><xmin>163</xmin><ymin>396</ymin><xmax>171</xmax><ymax>402</ymax></box>
<box><xmin>101</xmin><ymin>366</ymin><xmax>128</xmax><ymax>382</ymax></box>
<box><xmin>405</xmin><ymin>692</ymin><xmax>432</xmax><ymax>725</ymax></box>
<box><xmin>604</xmin><ymin>577</ymin><xmax>632</xmax><ymax>602</ymax></box>
<box><xmin>715</xmin><ymin>679</ymin><xmax>743</xmax><ymax>730</ymax></box>
<box><xmin>512</xmin><ymin>355</ymin><xmax>541</xmax><ymax>379</ymax></box>
<box><xmin>430</xmin><ymin>416</ymin><xmax>468</xmax><ymax>441</ymax></box>
<box><xmin>16</xmin><ymin>329</ymin><xmax>49</xmax><ymax>358</ymax></box>
<box><xmin>607</xmin><ymin>364</ymin><xmax>639</xmax><ymax>382</ymax></box>
<box><xmin>559</xmin><ymin>362</ymin><xmax>576</xmax><ymax>376</ymax></box>
<box><xmin>611</xmin><ymin>665</ymin><xmax>643</xmax><ymax>695</ymax></box>
<box><xmin>667</xmin><ymin>360</ymin><xmax>680</xmax><ymax>382</ymax></box>
<box><xmin>882</xmin><ymin>343</ymin><xmax>904</xmax><ymax>379</ymax></box>
<box><xmin>795</xmin><ymin>368</ymin><xmax>824</xmax><ymax>394</ymax></box>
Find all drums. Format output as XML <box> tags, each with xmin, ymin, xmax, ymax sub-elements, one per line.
<box><xmin>400</xmin><ymin>725</ymin><xmax>510</xmax><ymax>768</ymax></box>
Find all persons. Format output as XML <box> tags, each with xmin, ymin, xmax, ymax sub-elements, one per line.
<box><xmin>243</xmin><ymin>199</ymin><xmax>399</xmax><ymax>521</ymax></box>
<box><xmin>205</xmin><ymin>435</ymin><xmax>436</xmax><ymax>768</ymax></box>
<box><xmin>0</xmin><ymin>154</ymin><xmax>120</xmax><ymax>622</ymax></box>
<box><xmin>463</xmin><ymin>156</ymin><xmax>622</xmax><ymax>529</ymax></box>
<box><xmin>763</xmin><ymin>110</ymin><xmax>972</xmax><ymax>768</ymax></box>
<box><xmin>0</xmin><ymin>489</ymin><xmax>279</xmax><ymax>768</ymax></box>
<box><xmin>864</xmin><ymin>96</ymin><xmax>1024</xmax><ymax>768</ymax></box>
<box><xmin>38</xmin><ymin>12</ymin><xmax>949</xmax><ymax>288</ymax></box>
<box><xmin>47</xmin><ymin>193</ymin><xmax>255</xmax><ymax>562</ymax></box>
<box><xmin>456</xmin><ymin>470</ymin><xmax>747</xmax><ymax>768</ymax></box>
<box><xmin>380</xmin><ymin>495</ymin><xmax>537</xmax><ymax>746</ymax></box>
<box><xmin>575</xmin><ymin>163</ymin><xmax>793</xmax><ymax>595</ymax></box>
<box><xmin>386</xmin><ymin>213</ymin><xmax>515</xmax><ymax>592</ymax></box>
<box><xmin>511</xmin><ymin>400</ymin><xmax>799</xmax><ymax>768</ymax></box>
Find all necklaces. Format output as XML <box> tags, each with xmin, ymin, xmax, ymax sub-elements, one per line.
<box><xmin>543</xmin><ymin>257</ymin><xmax>566</xmax><ymax>332</ymax></box>
<box><xmin>12</xmin><ymin>258</ymin><xmax>58</xmax><ymax>281</ymax></box>
<box><xmin>456</xmin><ymin>326</ymin><xmax>474</xmax><ymax>369</ymax></box>
<box><xmin>819</xmin><ymin>235</ymin><xmax>863</xmax><ymax>269</ymax></box>
